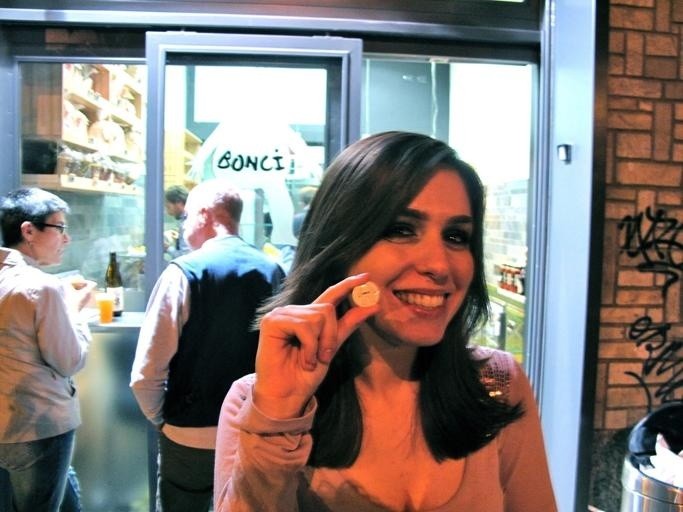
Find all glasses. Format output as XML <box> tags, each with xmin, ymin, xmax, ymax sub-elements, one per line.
<box><xmin>29</xmin><ymin>221</ymin><xmax>69</xmax><ymax>236</ymax></box>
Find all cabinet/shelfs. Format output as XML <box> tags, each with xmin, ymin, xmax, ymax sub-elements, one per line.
<box><xmin>18</xmin><ymin>61</ymin><xmax>202</xmax><ymax>195</ymax></box>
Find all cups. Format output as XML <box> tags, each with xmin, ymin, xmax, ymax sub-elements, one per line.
<box><xmin>96</xmin><ymin>292</ymin><xmax>114</xmax><ymax>324</ymax></box>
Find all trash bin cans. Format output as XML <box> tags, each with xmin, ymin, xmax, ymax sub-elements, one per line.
<box><xmin>617</xmin><ymin>400</ymin><xmax>683</xmax><ymax>512</ymax></box>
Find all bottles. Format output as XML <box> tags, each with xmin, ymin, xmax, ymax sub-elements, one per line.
<box><xmin>104</xmin><ymin>251</ymin><xmax>125</xmax><ymax>316</ymax></box>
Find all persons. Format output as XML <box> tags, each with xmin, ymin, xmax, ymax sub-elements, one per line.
<box><xmin>211</xmin><ymin>130</ymin><xmax>559</xmax><ymax>512</ymax></box>
<box><xmin>128</xmin><ymin>177</ymin><xmax>286</xmax><ymax>512</ymax></box>
<box><xmin>1</xmin><ymin>186</ymin><xmax>99</xmax><ymax>512</ymax></box>
<box><xmin>162</xmin><ymin>184</ymin><xmax>192</xmax><ymax>260</ymax></box>
<box><xmin>292</xmin><ymin>186</ymin><xmax>318</xmax><ymax>237</ymax></box>
